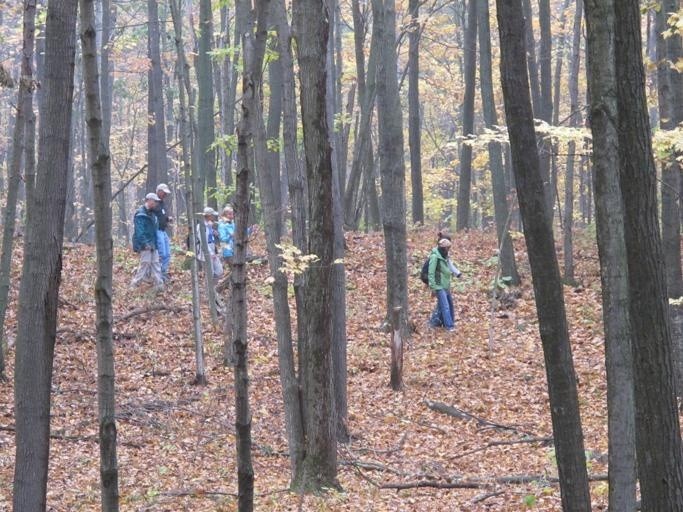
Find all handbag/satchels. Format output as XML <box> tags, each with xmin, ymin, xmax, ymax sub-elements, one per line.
<box><xmin>421</xmin><ymin>261</ymin><xmax>429</xmax><ymax>283</ymax></box>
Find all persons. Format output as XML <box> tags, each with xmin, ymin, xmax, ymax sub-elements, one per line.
<box><xmin>152</xmin><ymin>183</ymin><xmax>173</xmax><ymax>283</ymax></box>
<box><xmin>129</xmin><ymin>193</ymin><xmax>165</xmax><ymax>292</ymax></box>
<box><xmin>427</xmin><ymin>232</ymin><xmax>461</xmax><ymax>331</ymax></box>
<box><xmin>216</xmin><ymin>205</ymin><xmax>259</xmax><ymax>291</ymax></box>
<box><xmin>192</xmin><ymin>208</ymin><xmax>224</xmax><ymax>284</ymax></box>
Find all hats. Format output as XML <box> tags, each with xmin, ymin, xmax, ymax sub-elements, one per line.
<box><xmin>204</xmin><ymin>207</ymin><xmax>218</xmax><ymax>216</ymax></box>
<box><xmin>439</xmin><ymin>239</ymin><xmax>451</xmax><ymax>247</ymax></box>
<box><xmin>147</xmin><ymin>193</ymin><xmax>161</xmax><ymax>201</ymax></box>
<box><xmin>157</xmin><ymin>183</ymin><xmax>170</xmax><ymax>193</ymax></box>
